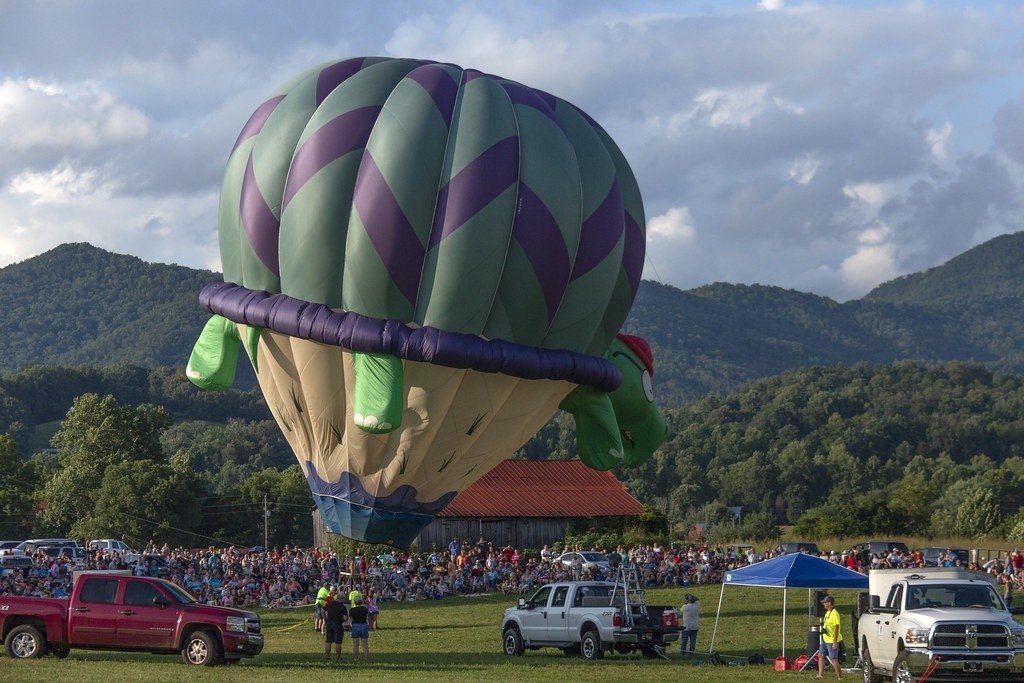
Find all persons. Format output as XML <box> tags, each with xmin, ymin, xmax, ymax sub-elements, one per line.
<box><xmin>0</xmin><ymin>534</ymin><xmax>926</xmax><ymax>607</ymax></box>
<box><xmin>938</xmin><ymin>549</ymin><xmax>966</xmax><ymax>569</ymax></box>
<box><xmin>680</xmin><ymin>593</ymin><xmax>700</xmax><ymax>657</ymax></box>
<box><xmin>969</xmin><ymin>562</ymin><xmax>982</xmax><ymax>571</ymax></box>
<box><xmin>814</xmin><ymin>596</ymin><xmax>845</xmax><ymax>680</ymax></box>
<box><xmin>989</xmin><ymin>548</ymin><xmax>1024</xmax><ymax>613</ymax></box>
<box><xmin>314</xmin><ymin>583</ymin><xmax>379</xmax><ymax>662</ymax></box>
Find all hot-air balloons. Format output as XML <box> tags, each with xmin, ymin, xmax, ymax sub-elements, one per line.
<box><xmin>183</xmin><ymin>51</ymin><xmax>677</xmax><ymax>619</ymax></box>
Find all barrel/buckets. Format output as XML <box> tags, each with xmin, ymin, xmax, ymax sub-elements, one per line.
<box><xmin>794</xmin><ymin>655</ymin><xmax>818</xmax><ymax>670</ymax></box>
<box><xmin>663</xmin><ymin>610</ymin><xmax>678</xmax><ymax>626</ymax></box>
<box><xmin>775</xmin><ymin>656</ymin><xmax>791</xmax><ymax>672</ymax></box>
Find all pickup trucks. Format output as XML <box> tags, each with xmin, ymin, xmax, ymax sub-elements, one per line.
<box><xmin>500</xmin><ymin>581</ymin><xmax>687</xmax><ymax>661</ymax></box>
<box><xmin>857</xmin><ymin>574</ymin><xmax>1024</xmax><ymax>683</ymax></box>
<box><xmin>0</xmin><ymin>572</ymin><xmax>265</xmax><ymax>667</ymax></box>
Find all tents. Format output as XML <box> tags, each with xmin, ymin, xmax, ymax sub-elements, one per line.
<box><xmin>710</xmin><ymin>554</ymin><xmax>869</xmax><ymax>660</ymax></box>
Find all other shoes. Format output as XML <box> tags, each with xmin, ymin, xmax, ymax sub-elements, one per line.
<box><xmin>836</xmin><ymin>676</ymin><xmax>843</xmax><ymax>681</ymax></box>
<box><xmin>813</xmin><ymin>675</ymin><xmax>824</xmax><ymax>679</ymax></box>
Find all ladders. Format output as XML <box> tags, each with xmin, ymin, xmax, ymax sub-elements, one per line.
<box><xmin>609</xmin><ymin>560</ymin><xmax>650</xmax><ymax>627</ymax></box>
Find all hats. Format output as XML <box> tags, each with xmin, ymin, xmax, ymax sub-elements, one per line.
<box><xmin>336</xmin><ymin>593</ymin><xmax>348</xmax><ymax>599</ymax></box>
<box><xmin>820</xmin><ymin>596</ymin><xmax>834</xmax><ymax>604</ymax></box>
<box><xmin>324</xmin><ymin>583</ymin><xmax>332</xmax><ymax>586</ymax></box>
<box><xmin>354</xmin><ymin>596</ymin><xmax>362</xmax><ymax>604</ymax></box>
<box><xmin>685</xmin><ymin>593</ymin><xmax>696</xmax><ymax>601</ymax></box>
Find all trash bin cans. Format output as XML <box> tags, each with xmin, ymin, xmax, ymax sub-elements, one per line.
<box><xmin>807</xmin><ymin>625</ymin><xmax>820</xmax><ymax>661</ymax></box>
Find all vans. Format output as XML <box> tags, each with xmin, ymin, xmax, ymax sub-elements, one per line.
<box><xmin>849</xmin><ymin>541</ymin><xmax>910</xmax><ymax>563</ymax></box>
<box><xmin>774</xmin><ymin>542</ymin><xmax>821</xmax><ymax>558</ymax></box>
<box><xmin>917</xmin><ymin>546</ymin><xmax>969</xmax><ymax>568</ymax></box>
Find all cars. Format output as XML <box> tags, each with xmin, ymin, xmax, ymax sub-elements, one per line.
<box><xmin>553</xmin><ymin>550</ymin><xmax>611</xmax><ymax>571</ymax></box>
<box><xmin>0</xmin><ymin>538</ymin><xmax>137</xmax><ymax>584</ymax></box>
